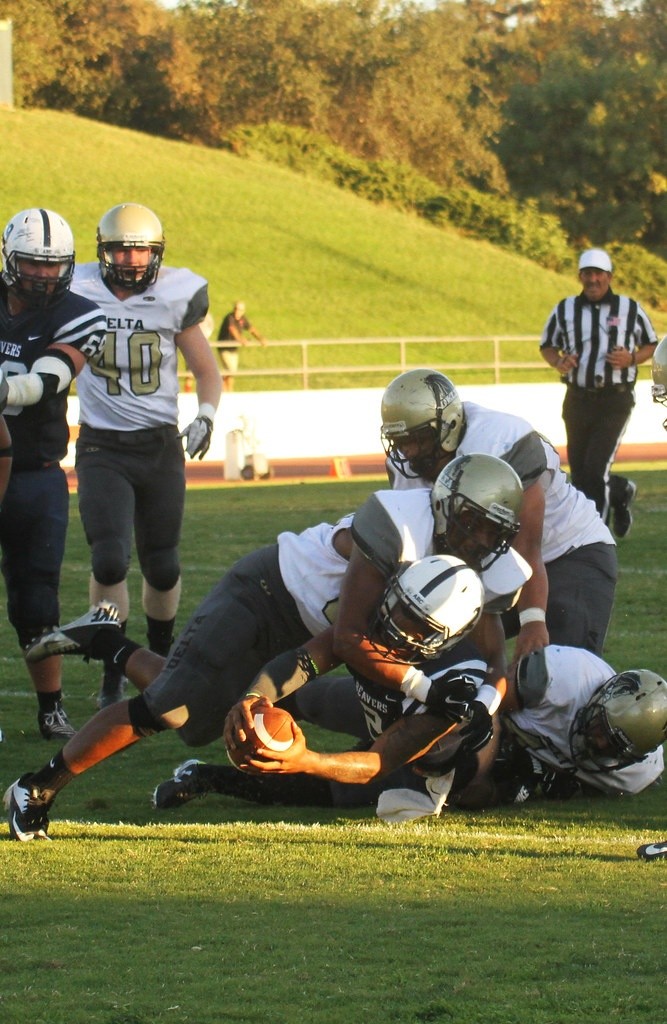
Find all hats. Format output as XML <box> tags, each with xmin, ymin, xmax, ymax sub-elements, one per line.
<box><xmin>579</xmin><ymin>249</ymin><xmax>612</xmax><ymax>272</ymax></box>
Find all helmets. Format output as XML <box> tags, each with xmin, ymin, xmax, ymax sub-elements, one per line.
<box><xmin>2</xmin><ymin>209</ymin><xmax>73</xmax><ymax>286</ymax></box>
<box><xmin>586</xmin><ymin>669</ymin><xmax>667</xmax><ymax>770</ymax></box>
<box><xmin>651</xmin><ymin>337</ymin><xmax>667</xmax><ymax>395</ymax></box>
<box><xmin>430</xmin><ymin>453</ymin><xmax>524</xmax><ymax>534</ymax></box>
<box><xmin>96</xmin><ymin>204</ymin><xmax>162</xmax><ymax>278</ymax></box>
<box><xmin>381</xmin><ymin>369</ymin><xmax>463</xmax><ymax>452</ymax></box>
<box><xmin>381</xmin><ymin>554</ymin><xmax>485</xmax><ymax>660</ymax></box>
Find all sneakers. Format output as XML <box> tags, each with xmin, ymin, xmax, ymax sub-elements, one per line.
<box><xmin>26</xmin><ymin>599</ymin><xmax>121</xmax><ymax>664</ymax></box>
<box><xmin>636</xmin><ymin>840</ymin><xmax>667</xmax><ymax>861</ymax></box>
<box><xmin>96</xmin><ymin>670</ymin><xmax>129</xmax><ymax>710</ymax></box>
<box><xmin>613</xmin><ymin>480</ymin><xmax>637</xmax><ymax>538</ymax></box>
<box><xmin>150</xmin><ymin>759</ymin><xmax>216</xmax><ymax>810</ymax></box>
<box><xmin>3</xmin><ymin>772</ymin><xmax>53</xmax><ymax>842</ymax></box>
<box><xmin>37</xmin><ymin>702</ymin><xmax>78</xmax><ymax>739</ymax></box>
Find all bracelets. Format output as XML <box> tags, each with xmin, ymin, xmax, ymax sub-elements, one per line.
<box><xmin>243</xmin><ymin>690</ymin><xmax>262</xmax><ymax>698</ymax></box>
<box><xmin>631</xmin><ymin>354</ymin><xmax>635</xmax><ymax>366</ymax></box>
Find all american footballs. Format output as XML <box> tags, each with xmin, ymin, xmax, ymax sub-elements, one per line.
<box><xmin>225</xmin><ymin>705</ymin><xmax>297</xmax><ymax>775</ymax></box>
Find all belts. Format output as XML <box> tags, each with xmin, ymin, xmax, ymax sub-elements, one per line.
<box><xmin>564</xmin><ymin>380</ymin><xmax>628</xmax><ymax>393</ymax></box>
<box><xmin>79</xmin><ymin>424</ymin><xmax>178</xmax><ymax>444</ymax></box>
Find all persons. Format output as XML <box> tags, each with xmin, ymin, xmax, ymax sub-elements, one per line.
<box><xmin>0</xmin><ymin>368</ymin><xmax>667</xmax><ymax>844</ymax></box>
<box><xmin>539</xmin><ymin>249</ymin><xmax>658</xmax><ymax>539</ymax></box>
<box><xmin>215</xmin><ymin>300</ymin><xmax>268</xmax><ymax>393</ymax></box>
<box><xmin>0</xmin><ymin>201</ymin><xmax>107</xmax><ymax>741</ymax></box>
<box><xmin>64</xmin><ymin>204</ymin><xmax>224</xmax><ymax>711</ymax></box>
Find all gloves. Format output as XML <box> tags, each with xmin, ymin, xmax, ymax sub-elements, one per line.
<box><xmin>425</xmin><ymin>674</ymin><xmax>494</xmax><ymax>754</ymax></box>
<box><xmin>176</xmin><ymin>415</ymin><xmax>213</xmax><ymax>459</ymax></box>
